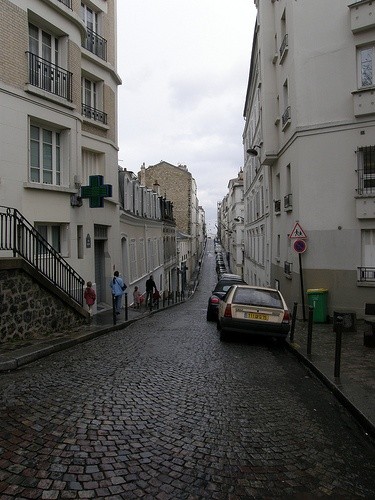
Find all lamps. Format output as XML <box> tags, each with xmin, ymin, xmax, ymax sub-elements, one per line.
<box><xmin>246</xmin><ymin>145</ymin><xmax>261</xmax><ymax>156</ymax></box>
<box><xmin>234</xmin><ymin>215</ymin><xmax>244</xmax><ymax>222</ymax></box>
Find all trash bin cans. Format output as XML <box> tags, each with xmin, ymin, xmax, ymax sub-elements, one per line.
<box><xmin>305</xmin><ymin>287</ymin><xmax>331</xmax><ymax>325</ymax></box>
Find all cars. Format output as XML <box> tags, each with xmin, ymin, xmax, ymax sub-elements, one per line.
<box><xmin>206</xmin><ymin>278</ymin><xmax>249</xmax><ymax>320</ymax></box>
<box><xmin>217</xmin><ymin>286</ymin><xmax>291</xmax><ymax>342</ymax></box>
<box><xmin>213</xmin><ymin>237</ymin><xmax>228</xmax><ymax>276</ymax></box>
<box><xmin>220</xmin><ymin>273</ymin><xmax>242</xmax><ymax>280</ymax></box>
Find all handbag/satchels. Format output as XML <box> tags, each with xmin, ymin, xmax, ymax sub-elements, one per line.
<box><xmin>121</xmin><ymin>284</ymin><xmax>127</xmax><ymax>291</ymax></box>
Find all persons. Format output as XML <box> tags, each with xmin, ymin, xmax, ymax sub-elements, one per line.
<box><xmin>146</xmin><ymin>276</ymin><xmax>161</xmax><ymax>306</ymax></box>
<box><xmin>110</xmin><ymin>271</ymin><xmax>124</xmax><ymax>314</ymax></box>
<box><xmin>133</xmin><ymin>286</ymin><xmax>140</xmax><ymax>309</ymax></box>
<box><xmin>84</xmin><ymin>281</ymin><xmax>97</xmax><ymax>317</ymax></box>
